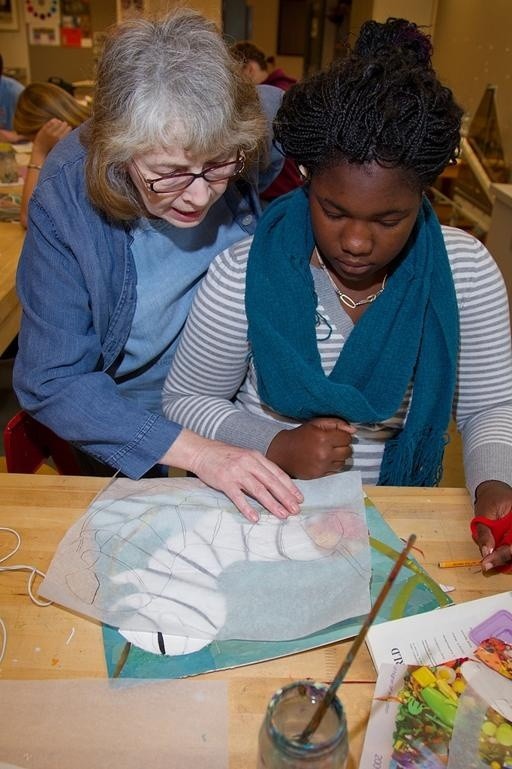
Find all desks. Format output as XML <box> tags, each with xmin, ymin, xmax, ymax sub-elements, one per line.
<box><xmin>1</xmin><ymin>473</ymin><xmax>512</xmax><ymax>769</ymax></box>
<box><xmin>0</xmin><ymin>220</ymin><xmax>43</xmax><ymax>357</ymax></box>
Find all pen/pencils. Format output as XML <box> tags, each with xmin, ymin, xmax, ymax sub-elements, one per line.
<box><xmin>438</xmin><ymin>560</ymin><xmax>482</xmax><ymax>566</ymax></box>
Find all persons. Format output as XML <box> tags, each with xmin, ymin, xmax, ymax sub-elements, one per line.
<box><xmin>8</xmin><ymin>8</ymin><xmax>310</xmax><ymax>525</ymax></box>
<box><xmin>0</xmin><ymin>55</ymin><xmax>37</xmax><ymax>145</ymax></box>
<box><xmin>227</xmin><ymin>42</ymin><xmax>298</xmax><ymax>92</ymax></box>
<box><xmin>156</xmin><ymin>13</ymin><xmax>512</xmax><ymax>576</ymax></box>
<box><xmin>12</xmin><ymin>82</ymin><xmax>93</xmax><ymax>230</ymax></box>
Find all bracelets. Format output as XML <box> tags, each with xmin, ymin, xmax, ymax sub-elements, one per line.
<box><xmin>28</xmin><ymin>163</ymin><xmax>42</xmax><ymax>170</ymax></box>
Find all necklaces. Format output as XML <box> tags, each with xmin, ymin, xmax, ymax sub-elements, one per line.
<box><xmin>312</xmin><ymin>245</ymin><xmax>394</xmax><ymax>311</ymax></box>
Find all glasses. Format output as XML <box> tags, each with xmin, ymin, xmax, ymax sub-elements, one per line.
<box><xmin>129</xmin><ymin>149</ymin><xmax>245</xmax><ymax>194</ymax></box>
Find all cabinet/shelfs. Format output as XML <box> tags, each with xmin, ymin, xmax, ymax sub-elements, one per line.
<box><xmin>485</xmin><ymin>182</ymin><xmax>512</xmax><ymax>306</ymax></box>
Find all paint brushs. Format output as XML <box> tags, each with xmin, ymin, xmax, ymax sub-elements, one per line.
<box><xmin>297</xmin><ymin>533</ymin><xmax>418</xmax><ymax>744</ymax></box>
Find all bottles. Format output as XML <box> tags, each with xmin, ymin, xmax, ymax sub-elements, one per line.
<box><xmin>258</xmin><ymin>681</ymin><xmax>350</xmax><ymax>769</ymax></box>
